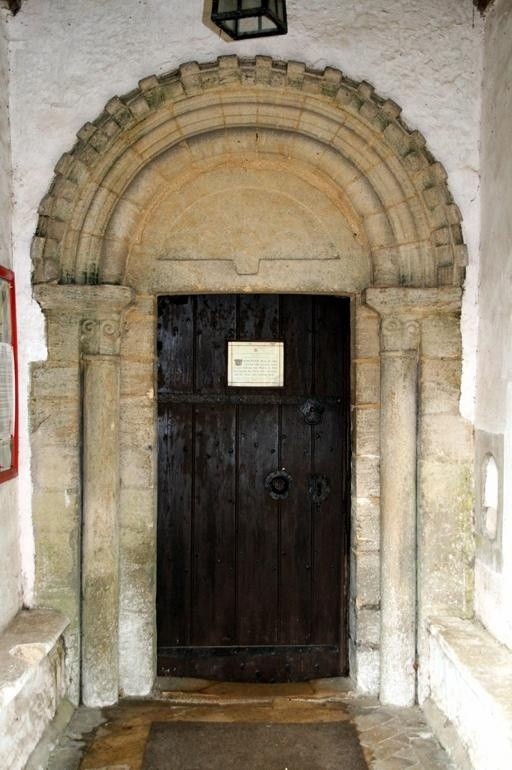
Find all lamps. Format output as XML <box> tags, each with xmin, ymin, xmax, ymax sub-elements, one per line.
<box><xmin>211</xmin><ymin>0</ymin><xmax>287</xmax><ymax>41</ymax></box>
